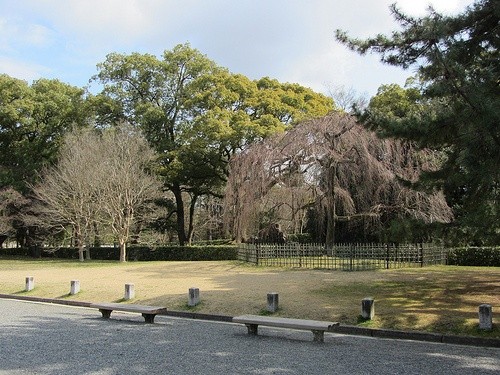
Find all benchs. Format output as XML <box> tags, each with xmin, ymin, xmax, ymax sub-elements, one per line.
<box><xmin>232</xmin><ymin>314</ymin><xmax>340</xmax><ymax>343</ymax></box>
<box><xmin>90</xmin><ymin>302</ymin><xmax>167</xmax><ymax>323</ymax></box>
